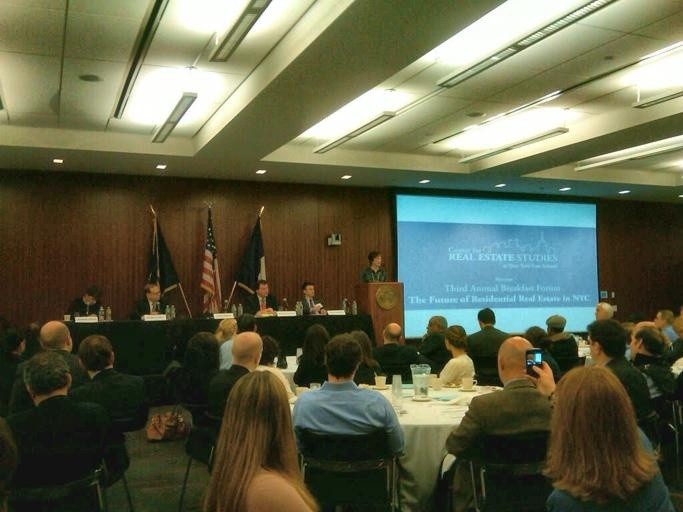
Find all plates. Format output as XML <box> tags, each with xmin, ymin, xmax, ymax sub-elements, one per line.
<box><xmin>413</xmin><ymin>396</ymin><xmax>430</xmax><ymax>401</ymax></box>
<box><xmin>371</xmin><ymin>387</ymin><xmax>388</xmax><ymax>389</ymax></box>
<box><xmin>61</xmin><ymin>320</ymin><xmax>72</xmax><ymax>322</ymax></box>
<box><xmin>461</xmin><ymin>387</ymin><xmax>479</xmax><ymax>391</ymax></box>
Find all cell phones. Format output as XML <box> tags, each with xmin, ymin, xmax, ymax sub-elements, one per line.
<box><xmin>526</xmin><ymin>348</ymin><xmax>543</xmax><ymax>377</ymax></box>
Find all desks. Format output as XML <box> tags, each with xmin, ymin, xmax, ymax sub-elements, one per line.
<box><xmin>60</xmin><ymin>314</ymin><xmax>367</xmax><ymax>376</ymax></box>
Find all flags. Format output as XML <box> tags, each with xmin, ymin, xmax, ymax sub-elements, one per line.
<box><xmin>198</xmin><ymin>209</ymin><xmax>224</xmax><ymax>315</ymax></box>
<box><xmin>145</xmin><ymin>218</ymin><xmax>179</xmax><ymax>297</ymax></box>
<box><xmin>233</xmin><ymin>215</ymin><xmax>271</xmax><ymax>296</ymax></box>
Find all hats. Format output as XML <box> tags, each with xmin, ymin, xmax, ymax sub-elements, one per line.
<box><xmin>546</xmin><ymin>314</ymin><xmax>566</xmax><ymax>329</ymax></box>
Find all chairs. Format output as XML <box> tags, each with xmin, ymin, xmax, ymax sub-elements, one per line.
<box><xmin>0</xmin><ymin>333</ymin><xmax>683</xmax><ymax>512</ymax></box>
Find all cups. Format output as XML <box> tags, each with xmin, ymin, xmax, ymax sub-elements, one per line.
<box><xmin>410</xmin><ymin>363</ymin><xmax>431</xmax><ymax>396</ymax></box>
<box><xmin>428</xmin><ymin>373</ymin><xmax>437</xmax><ymax>387</ymax></box>
<box><xmin>431</xmin><ymin>377</ymin><xmax>443</xmax><ymax>389</ymax></box>
<box><xmin>375</xmin><ymin>376</ymin><xmax>386</xmax><ymax>388</ymax></box>
<box><xmin>63</xmin><ymin>315</ymin><xmax>70</xmax><ymax>320</ymax></box>
<box><xmin>295</xmin><ymin>386</ymin><xmax>308</xmax><ymax>398</ymax></box>
<box><xmin>462</xmin><ymin>377</ymin><xmax>477</xmax><ymax>390</ymax></box>
<box><xmin>309</xmin><ymin>382</ymin><xmax>319</xmax><ymax>389</ymax></box>
<box><xmin>579</xmin><ymin>340</ymin><xmax>587</xmax><ymax>347</ymax></box>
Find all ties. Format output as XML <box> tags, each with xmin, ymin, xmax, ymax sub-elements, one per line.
<box><xmin>310</xmin><ymin>299</ymin><xmax>313</xmax><ymax>307</ymax></box>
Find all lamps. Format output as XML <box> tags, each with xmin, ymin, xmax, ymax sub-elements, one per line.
<box><xmin>149</xmin><ymin>89</ymin><xmax>197</xmax><ymax>143</ymax></box>
<box><xmin>312</xmin><ymin>96</ymin><xmax>396</xmax><ymax>155</ymax></box>
<box><xmin>435</xmin><ymin>0</ymin><xmax>622</xmax><ymax>89</ymax></box>
<box><xmin>633</xmin><ymin>79</ymin><xmax>683</xmax><ymax>109</ymax></box>
<box><xmin>459</xmin><ymin>110</ymin><xmax>569</xmax><ymax>166</ymax></box>
<box><xmin>209</xmin><ymin>0</ymin><xmax>272</xmax><ymax>62</ymax></box>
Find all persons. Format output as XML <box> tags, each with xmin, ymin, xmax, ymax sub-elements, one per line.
<box><xmin>466</xmin><ymin>307</ymin><xmax>513</xmax><ymax>387</ymax></box>
<box><xmin>585</xmin><ymin>319</ymin><xmax>658</xmax><ymax>450</ymax></box>
<box><xmin>299</xmin><ymin>281</ymin><xmax>326</xmax><ymax>315</ymax></box>
<box><xmin>358</xmin><ymin>251</ymin><xmax>388</xmax><ymax>282</ymax></box>
<box><xmin>446</xmin><ymin>336</ymin><xmax>555</xmax><ymax>512</ymax></box>
<box><xmin>127</xmin><ymin>281</ymin><xmax>169</xmax><ymax>320</ymax></box>
<box><xmin>292</xmin><ymin>333</ymin><xmax>405</xmax><ymax>512</ymax></box>
<box><xmin>524</xmin><ymin>314</ymin><xmax>579</xmax><ymax>384</ymax></box>
<box><xmin>418</xmin><ymin>316</ymin><xmax>453</xmax><ymax>378</ymax></box>
<box><xmin>538</xmin><ymin>362</ymin><xmax>681</xmax><ymax>510</ymax></box>
<box><xmin>62</xmin><ymin>285</ymin><xmax>105</xmax><ymax>322</ymax></box>
<box><xmin>439</xmin><ymin>325</ymin><xmax>475</xmax><ymax>384</ymax></box>
<box><xmin>594</xmin><ymin>302</ymin><xmax>681</xmax><ymax>421</ymax></box>
<box><xmin>294</xmin><ymin>323</ymin><xmax>419</xmax><ymax>388</ymax></box>
<box><xmin>243</xmin><ymin>278</ymin><xmax>280</xmax><ymax>317</ymax></box>
<box><xmin>197</xmin><ymin>369</ymin><xmax>322</xmax><ymax>511</ymax></box>
<box><xmin>0</xmin><ymin>320</ymin><xmax>152</xmax><ymax>511</ymax></box>
<box><xmin>163</xmin><ymin>313</ymin><xmax>295</xmax><ymax>475</ymax></box>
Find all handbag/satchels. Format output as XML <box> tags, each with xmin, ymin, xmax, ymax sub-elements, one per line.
<box><xmin>146</xmin><ymin>407</ymin><xmax>188</xmax><ymax>442</ymax></box>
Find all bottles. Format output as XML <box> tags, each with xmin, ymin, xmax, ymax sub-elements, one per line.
<box><xmin>237</xmin><ymin>304</ymin><xmax>241</xmax><ymax>317</ymax></box>
<box><xmin>232</xmin><ymin>304</ymin><xmax>236</xmax><ymax>317</ymax></box>
<box><xmin>295</xmin><ymin>301</ymin><xmax>303</xmax><ymax>316</ymax></box>
<box><xmin>343</xmin><ymin>296</ymin><xmax>350</xmax><ymax>314</ymax></box>
<box><xmin>98</xmin><ymin>306</ymin><xmax>104</xmax><ymax>321</ymax></box>
<box><xmin>165</xmin><ymin>305</ymin><xmax>170</xmax><ymax>320</ymax></box>
<box><xmin>351</xmin><ymin>300</ymin><xmax>357</xmax><ymax>315</ymax></box>
<box><xmin>170</xmin><ymin>305</ymin><xmax>175</xmax><ymax>320</ymax></box>
<box><xmin>281</xmin><ymin>298</ymin><xmax>287</xmax><ymax>310</ymax></box>
<box><xmin>391</xmin><ymin>374</ymin><xmax>402</xmax><ymax>410</ymax></box>
<box><xmin>105</xmin><ymin>306</ymin><xmax>110</xmax><ymax>320</ymax></box>
